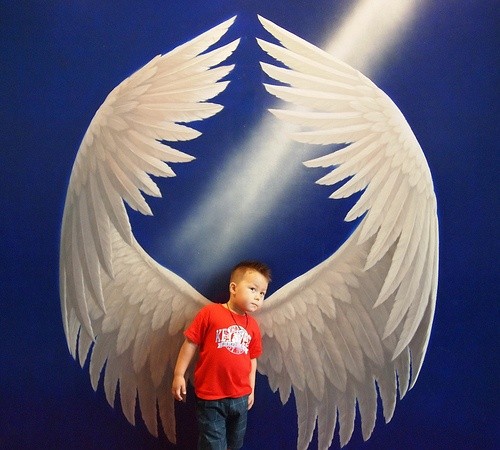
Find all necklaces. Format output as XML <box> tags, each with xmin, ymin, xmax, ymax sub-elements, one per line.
<box><xmin>227</xmin><ymin>303</ymin><xmax>248</xmax><ymax>335</ymax></box>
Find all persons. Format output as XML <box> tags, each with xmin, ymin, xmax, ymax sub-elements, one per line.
<box><xmin>171</xmin><ymin>259</ymin><xmax>271</xmax><ymax>449</ymax></box>
